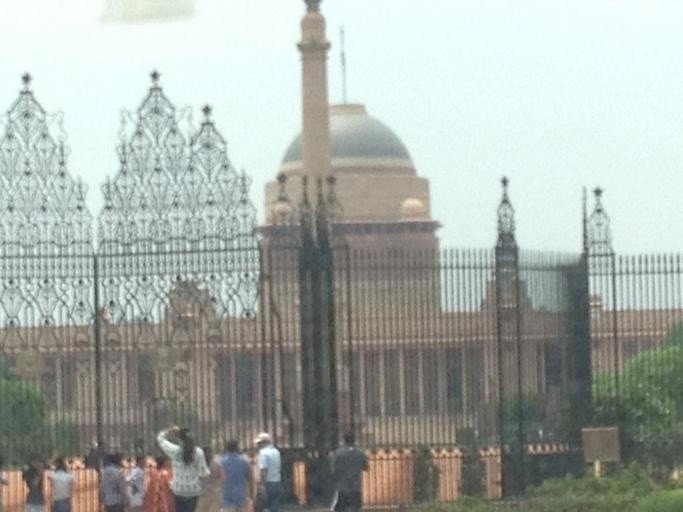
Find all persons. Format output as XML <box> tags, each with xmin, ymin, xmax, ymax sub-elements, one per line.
<box><xmin>22</xmin><ymin>455</ymin><xmax>75</xmax><ymax>512</ymax></box>
<box><xmin>98</xmin><ymin>425</ymin><xmax>282</xmax><ymax>512</ymax></box>
<box><xmin>329</xmin><ymin>430</ymin><xmax>370</xmax><ymax>512</ymax></box>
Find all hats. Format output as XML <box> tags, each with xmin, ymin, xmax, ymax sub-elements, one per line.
<box><xmin>254</xmin><ymin>432</ymin><xmax>272</xmax><ymax>444</ymax></box>
